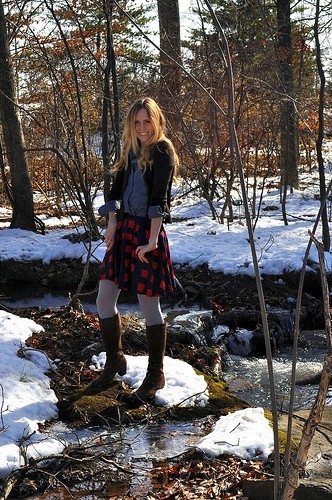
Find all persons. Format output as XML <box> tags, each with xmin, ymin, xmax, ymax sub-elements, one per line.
<box><xmin>91</xmin><ymin>99</ymin><xmax>180</xmax><ymax>403</ymax></box>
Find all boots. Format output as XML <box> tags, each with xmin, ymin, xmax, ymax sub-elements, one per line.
<box><xmin>88</xmin><ymin>312</ymin><xmax>127</xmax><ymax>387</ymax></box>
<box><xmin>132</xmin><ymin>320</ymin><xmax>167</xmax><ymax>399</ymax></box>
